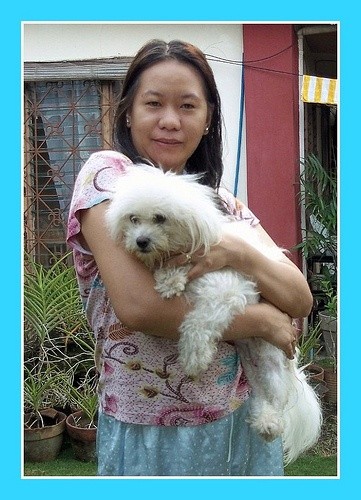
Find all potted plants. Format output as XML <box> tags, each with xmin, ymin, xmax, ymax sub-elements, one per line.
<box><xmin>294</xmin><ymin>156</ymin><xmax>338</xmax><ymax>405</ymax></box>
<box><xmin>24</xmin><ymin>249</ymin><xmax>99</xmax><ymax>462</ymax></box>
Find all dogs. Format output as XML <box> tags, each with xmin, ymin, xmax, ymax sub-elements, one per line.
<box><xmin>105</xmin><ymin>164</ymin><xmax>320</xmax><ymax>464</ymax></box>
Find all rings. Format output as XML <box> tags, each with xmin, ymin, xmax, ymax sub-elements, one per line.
<box><xmin>186</xmin><ymin>253</ymin><xmax>191</xmax><ymax>263</ymax></box>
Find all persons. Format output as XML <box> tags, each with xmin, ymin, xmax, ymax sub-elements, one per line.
<box><xmin>66</xmin><ymin>39</ymin><xmax>314</xmax><ymax>477</ymax></box>
